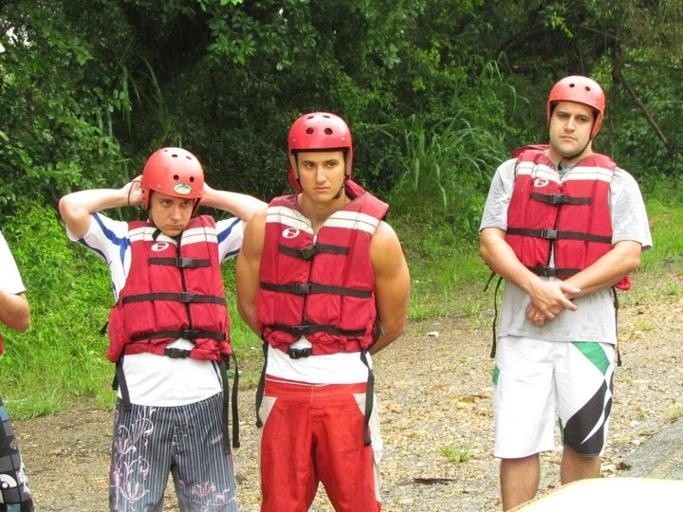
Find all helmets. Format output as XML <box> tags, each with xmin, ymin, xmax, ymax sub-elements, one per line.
<box><xmin>288</xmin><ymin>112</ymin><xmax>354</xmax><ymax>179</ymax></box>
<box><xmin>141</xmin><ymin>147</ymin><xmax>206</xmax><ymax>217</ymax></box>
<box><xmin>547</xmin><ymin>75</ymin><xmax>606</xmax><ymax>139</ymax></box>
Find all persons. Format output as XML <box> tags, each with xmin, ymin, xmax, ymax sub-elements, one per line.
<box><xmin>478</xmin><ymin>73</ymin><xmax>653</xmax><ymax>512</ymax></box>
<box><xmin>0</xmin><ymin>230</ymin><xmax>36</xmax><ymax>511</ymax></box>
<box><xmin>59</xmin><ymin>146</ymin><xmax>269</xmax><ymax>512</ymax></box>
<box><xmin>235</xmin><ymin>111</ymin><xmax>411</xmax><ymax>512</ymax></box>
